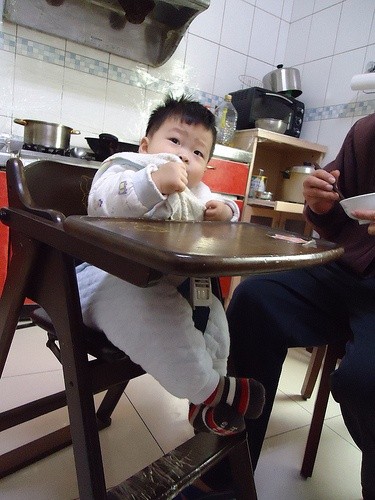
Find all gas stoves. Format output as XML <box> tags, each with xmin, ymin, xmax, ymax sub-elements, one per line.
<box><xmin>17</xmin><ymin>139</ymin><xmax>141</xmax><ymax>170</ymax></box>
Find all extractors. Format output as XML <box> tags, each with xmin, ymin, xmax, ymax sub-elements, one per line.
<box><xmin>0</xmin><ymin>0</ymin><xmax>212</xmax><ymax>68</ymax></box>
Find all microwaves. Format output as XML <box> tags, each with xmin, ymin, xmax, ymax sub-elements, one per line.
<box><xmin>229</xmin><ymin>86</ymin><xmax>305</xmax><ymax>137</ymax></box>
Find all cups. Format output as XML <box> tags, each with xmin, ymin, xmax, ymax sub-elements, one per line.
<box><xmin>249</xmin><ymin>175</ymin><xmax>268</xmax><ymax>198</ymax></box>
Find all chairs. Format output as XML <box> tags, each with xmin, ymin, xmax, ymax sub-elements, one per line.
<box><xmin>0</xmin><ymin>158</ymin><xmax>343</xmax><ymax>500</ymax></box>
<box><xmin>300</xmin><ymin>340</ymin><xmax>346</xmax><ymax>477</ymax></box>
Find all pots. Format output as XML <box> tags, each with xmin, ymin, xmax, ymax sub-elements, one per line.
<box><xmin>84</xmin><ymin>133</ymin><xmax>138</xmax><ymax>158</ymax></box>
<box><xmin>261</xmin><ymin>64</ymin><xmax>302</xmax><ymax>98</ymax></box>
<box><xmin>14</xmin><ymin>118</ymin><xmax>81</xmax><ymax>150</ymax></box>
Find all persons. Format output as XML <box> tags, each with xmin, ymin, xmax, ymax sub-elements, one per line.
<box><xmin>167</xmin><ymin>113</ymin><xmax>375</xmax><ymax>500</ymax></box>
<box><xmin>74</xmin><ymin>95</ymin><xmax>267</xmax><ymax>435</ymax></box>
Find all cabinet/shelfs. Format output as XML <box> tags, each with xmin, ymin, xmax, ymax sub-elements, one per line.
<box><xmin>231</xmin><ymin>128</ymin><xmax>328</xmax><ymax>235</ymax></box>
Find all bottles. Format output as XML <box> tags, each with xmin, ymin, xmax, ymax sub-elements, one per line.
<box><xmin>212</xmin><ymin>95</ymin><xmax>238</xmax><ymax>145</ymax></box>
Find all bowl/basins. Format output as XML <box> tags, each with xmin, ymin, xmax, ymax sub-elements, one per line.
<box><xmin>254</xmin><ymin>117</ymin><xmax>288</xmax><ymax>134</ymax></box>
<box><xmin>338</xmin><ymin>192</ymin><xmax>375</xmax><ymax>225</ymax></box>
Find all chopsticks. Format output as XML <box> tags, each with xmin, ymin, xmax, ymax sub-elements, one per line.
<box><xmin>312</xmin><ymin>160</ymin><xmax>345</xmax><ymax>200</ymax></box>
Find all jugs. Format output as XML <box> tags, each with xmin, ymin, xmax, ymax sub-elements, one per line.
<box><xmin>281</xmin><ymin>162</ymin><xmax>313</xmax><ymax>202</ymax></box>
